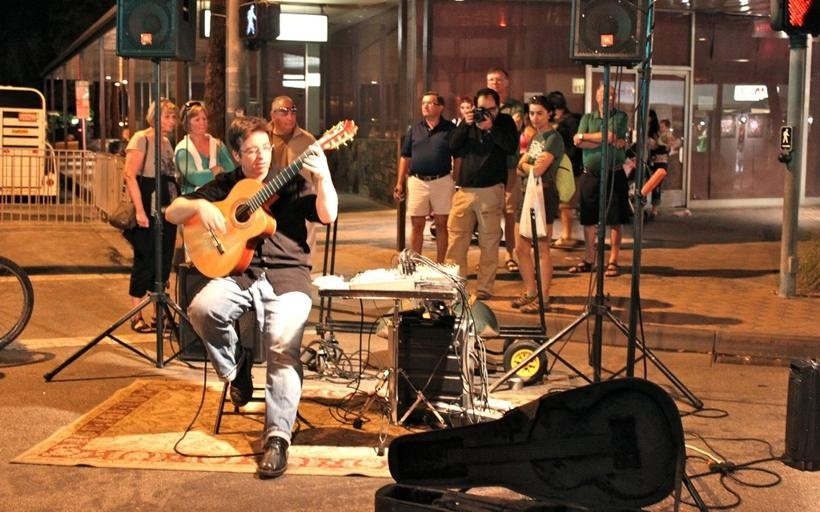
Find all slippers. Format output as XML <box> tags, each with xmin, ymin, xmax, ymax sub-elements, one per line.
<box><xmin>551</xmin><ymin>239</ymin><xmax>611</xmax><ymax>250</ymax></box>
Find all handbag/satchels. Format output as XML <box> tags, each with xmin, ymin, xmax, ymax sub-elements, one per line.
<box><xmin>554</xmin><ymin>153</ymin><xmax>576</xmax><ymax>202</ymax></box>
<box><xmin>109</xmin><ymin>201</ymin><xmax>137</xmax><ymax>230</ymax></box>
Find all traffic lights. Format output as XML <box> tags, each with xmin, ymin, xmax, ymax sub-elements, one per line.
<box><xmin>784</xmin><ymin>0</ymin><xmax>816</xmax><ymax>31</ymax></box>
<box><xmin>237</xmin><ymin>4</ymin><xmax>268</xmax><ymax>44</ymax></box>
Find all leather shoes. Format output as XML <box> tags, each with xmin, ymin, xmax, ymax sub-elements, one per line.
<box><xmin>258</xmin><ymin>436</ymin><xmax>287</xmax><ymax>476</ymax></box>
<box><xmin>231</xmin><ymin>348</ymin><xmax>253</xmax><ymax>407</ymax></box>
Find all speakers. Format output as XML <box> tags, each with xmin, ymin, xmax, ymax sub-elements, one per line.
<box><xmin>114</xmin><ymin>0</ymin><xmax>197</xmax><ymax>60</ymax></box>
<box><xmin>567</xmin><ymin>1</ymin><xmax>655</xmax><ymax>66</ymax></box>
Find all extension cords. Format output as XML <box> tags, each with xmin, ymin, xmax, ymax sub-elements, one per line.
<box><xmin>707</xmin><ymin>460</ymin><xmax>739</xmax><ymax>475</ymax></box>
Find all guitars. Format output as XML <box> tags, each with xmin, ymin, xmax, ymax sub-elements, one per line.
<box><xmin>182</xmin><ymin>119</ymin><xmax>359</xmax><ymax>278</ymax></box>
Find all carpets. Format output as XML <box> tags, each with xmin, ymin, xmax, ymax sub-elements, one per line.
<box><xmin>8</xmin><ymin>378</ymin><xmax>541</xmax><ymax>478</ymax></box>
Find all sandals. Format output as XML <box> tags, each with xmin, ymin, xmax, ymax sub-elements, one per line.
<box><xmin>130</xmin><ymin>314</ymin><xmax>178</xmax><ymax>333</ymax></box>
<box><xmin>569</xmin><ymin>260</ymin><xmax>619</xmax><ymax>276</ymax></box>
<box><xmin>505</xmin><ymin>259</ymin><xmax>518</xmax><ymax>273</ymax></box>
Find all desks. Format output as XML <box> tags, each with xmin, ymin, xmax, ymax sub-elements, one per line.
<box><xmin>319</xmin><ymin>287</ymin><xmax>460</xmax><ymax>425</ymax></box>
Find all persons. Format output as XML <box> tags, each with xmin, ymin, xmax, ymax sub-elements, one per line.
<box><xmin>393</xmin><ymin>92</ymin><xmax>462</xmax><ymax>265</ymax></box>
<box><xmin>569</xmin><ymin>82</ymin><xmax>628</xmax><ymax>279</ymax></box>
<box><xmin>164</xmin><ymin>117</ymin><xmax>338</xmax><ymax>479</ymax></box>
<box><xmin>450</xmin><ymin>66</ymin><xmax>583</xmax><ymax>253</ymax></box>
<box><xmin>173</xmin><ymin>100</ymin><xmax>236</xmax><ymax>263</ymax></box>
<box><xmin>515</xmin><ymin>95</ymin><xmax>564</xmax><ymax>312</ymax></box>
<box><xmin>124</xmin><ymin>96</ymin><xmax>180</xmax><ymax>334</ymax></box>
<box><xmin>451</xmin><ymin>89</ymin><xmax>519</xmax><ymax>297</ymax></box>
<box><xmin>263</xmin><ymin>96</ymin><xmax>320</xmax><ymax>270</ymax></box>
<box><xmin>621</xmin><ymin>108</ymin><xmax>683</xmax><ymax>223</ymax></box>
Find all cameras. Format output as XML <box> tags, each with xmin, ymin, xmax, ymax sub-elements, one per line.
<box><xmin>469</xmin><ymin>104</ymin><xmax>487</xmax><ymax>124</ymax></box>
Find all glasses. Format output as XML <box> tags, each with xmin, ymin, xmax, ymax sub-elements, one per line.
<box><xmin>421</xmin><ymin>102</ymin><xmax>439</xmax><ymax>106</ymax></box>
<box><xmin>181</xmin><ymin>101</ymin><xmax>201</xmax><ymax>120</ymax></box>
<box><xmin>238</xmin><ymin>144</ymin><xmax>276</xmax><ymax>156</ymax></box>
<box><xmin>274</xmin><ymin>106</ymin><xmax>298</xmax><ymax>112</ymax></box>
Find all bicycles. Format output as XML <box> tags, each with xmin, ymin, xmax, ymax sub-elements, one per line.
<box><xmin>0</xmin><ymin>253</ymin><xmax>35</xmax><ymax>355</ymax></box>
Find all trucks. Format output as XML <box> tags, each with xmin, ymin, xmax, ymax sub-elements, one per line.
<box><xmin>1</xmin><ymin>84</ymin><xmax>58</xmax><ymax>199</ymax></box>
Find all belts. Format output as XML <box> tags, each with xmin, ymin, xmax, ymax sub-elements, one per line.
<box><xmin>410</xmin><ymin>172</ymin><xmax>450</xmax><ymax>180</ymax></box>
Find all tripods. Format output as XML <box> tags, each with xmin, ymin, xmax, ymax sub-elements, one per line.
<box><xmin>42</xmin><ymin>59</ymin><xmax>208</xmax><ymax>383</ymax></box>
<box><xmin>353</xmin><ymin>316</ymin><xmax>447</xmax><ymax>456</ymax></box>
<box><xmin>486</xmin><ymin>66</ymin><xmax>710</xmax><ymax>412</ymax></box>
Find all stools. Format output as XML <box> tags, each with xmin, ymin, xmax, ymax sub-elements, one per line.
<box><xmin>213</xmin><ymin>307</ymin><xmax>318</xmax><ymax>434</ymax></box>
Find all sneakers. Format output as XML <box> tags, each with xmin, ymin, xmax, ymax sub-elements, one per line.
<box><xmin>476</xmin><ymin>290</ymin><xmax>490</xmax><ymax>299</ymax></box>
<box><xmin>512</xmin><ymin>294</ymin><xmax>551</xmax><ymax>313</ymax></box>
<box><xmin>642</xmin><ymin>210</ymin><xmax>657</xmax><ymax>221</ymax></box>
